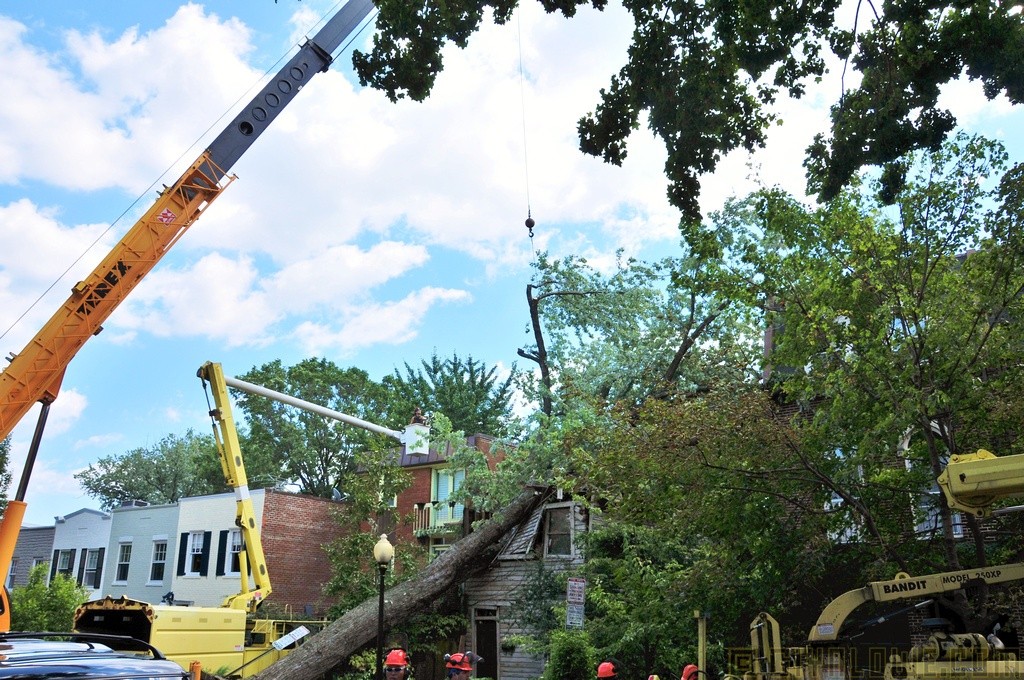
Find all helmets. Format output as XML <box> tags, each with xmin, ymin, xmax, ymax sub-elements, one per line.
<box><xmin>681</xmin><ymin>665</ymin><xmax>699</xmax><ymax>680</ymax></box>
<box><xmin>597</xmin><ymin>661</ymin><xmax>619</xmax><ymax>677</ymax></box>
<box><xmin>443</xmin><ymin>651</ymin><xmax>483</xmax><ymax>670</ymax></box>
<box><xmin>385</xmin><ymin>649</ymin><xmax>408</xmax><ymax>665</ymax></box>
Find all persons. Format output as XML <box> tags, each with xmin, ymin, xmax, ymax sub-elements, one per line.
<box><xmin>445</xmin><ymin>653</ymin><xmax>473</xmax><ymax>680</ymax></box>
<box><xmin>596</xmin><ymin>662</ymin><xmax>618</xmax><ymax>680</ymax></box>
<box><xmin>383</xmin><ymin>649</ymin><xmax>408</xmax><ymax>680</ymax></box>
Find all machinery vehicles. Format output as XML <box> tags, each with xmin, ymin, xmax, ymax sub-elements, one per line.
<box><xmin>693</xmin><ymin>448</ymin><xmax>1024</xmax><ymax>680</ymax></box>
<box><xmin>0</xmin><ymin>0</ymin><xmax>431</xmax><ymax>680</ymax></box>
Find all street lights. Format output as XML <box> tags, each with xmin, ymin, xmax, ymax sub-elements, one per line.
<box><xmin>372</xmin><ymin>534</ymin><xmax>393</xmax><ymax>680</ymax></box>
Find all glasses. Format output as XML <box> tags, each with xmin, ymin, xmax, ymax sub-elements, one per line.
<box><xmin>386</xmin><ymin>666</ymin><xmax>405</xmax><ymax>672</ymax></box>
